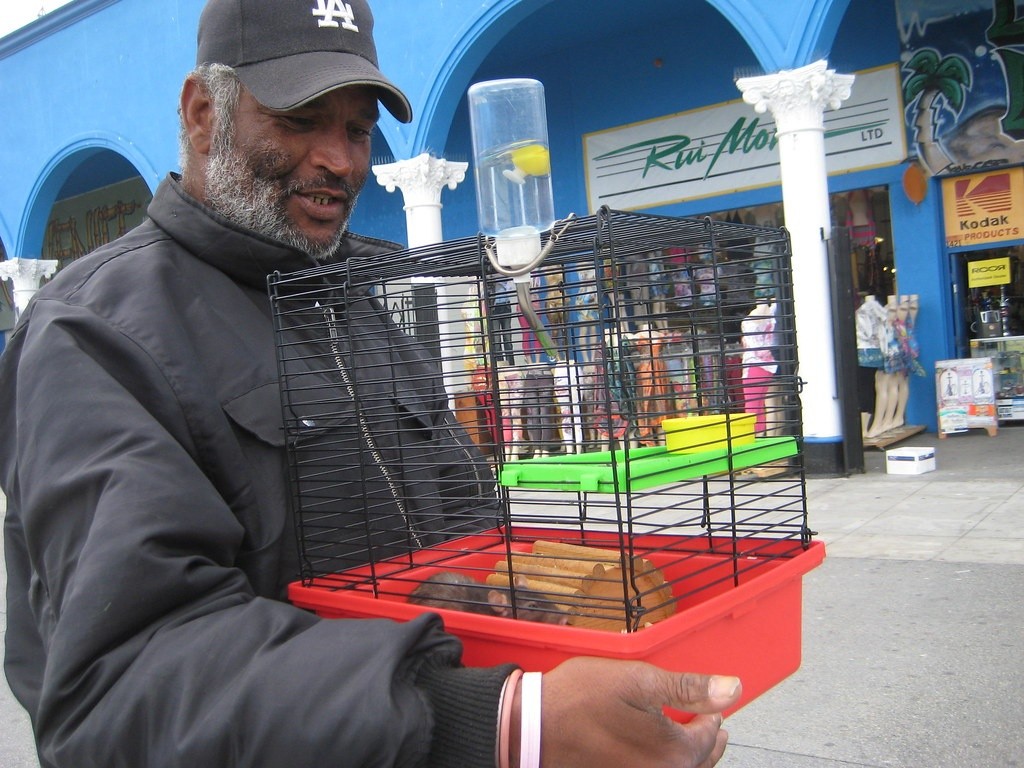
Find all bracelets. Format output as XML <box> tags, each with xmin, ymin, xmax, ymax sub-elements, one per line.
<box><xmin>500</xmin><ymin>669</ymin><xmax>543</xmax><ymax>768</ymax></box>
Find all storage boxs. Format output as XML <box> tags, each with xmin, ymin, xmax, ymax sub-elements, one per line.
<box><xmin>886</xmin><ymin>446</ymin><xmax>936</xmax><ymax>474</ymax></box>
<box><xmin>262</xmin><ymin>205</ymin><xmax>827</xmax><ymax>728</ymax></box>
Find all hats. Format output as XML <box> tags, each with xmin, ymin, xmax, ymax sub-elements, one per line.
<box><xmin>195</xmin><ymin>0</ymin><xmax>413</xmax><ymax>123</ymax></box>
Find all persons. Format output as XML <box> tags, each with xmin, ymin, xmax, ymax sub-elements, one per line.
<box><xmin>0</xmin><ymin>1</ymin><xmax>741</xmax><ymax>768</ymax></box>
<box><xmin>497</xmin><ymin>235</ymin><xmax>917</xmax><ymax>462</ymax></box>
